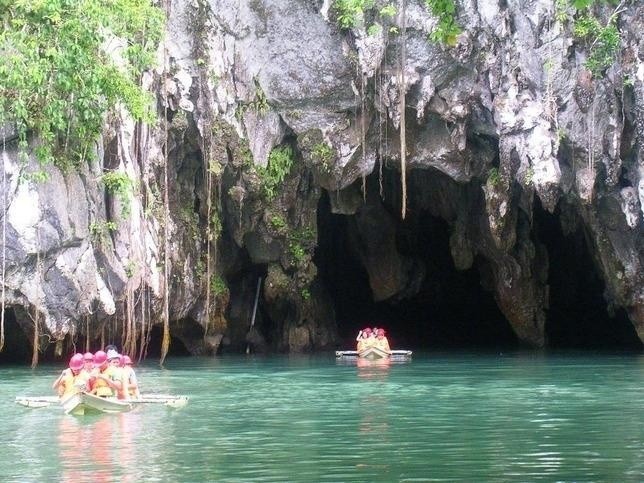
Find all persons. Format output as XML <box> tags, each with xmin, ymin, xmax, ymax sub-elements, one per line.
<box><xmin>52</xmin><ymin>342</ymin><xmax>141</xmax><ymax>401</ymax></box>
<box><xmin>354</xmin><ymin>327</ymin><xmax>390</xmax><ymax>350</ymax></box>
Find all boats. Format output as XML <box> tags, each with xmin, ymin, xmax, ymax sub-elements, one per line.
<box><xmin>60</xmin><ymin>388</ymin><xmax>142</xmax><ymax>416</ymax></box>
<box><xmin>358</xmin><ymin>345</ymin><xmax>392</xmax><ymax>361</ymax></box>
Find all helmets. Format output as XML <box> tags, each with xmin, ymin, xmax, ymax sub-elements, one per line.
<box><xmin>70</xmin><ymin>350</ymin><xmax>132</xmax><ymax>371</ymax></box>
<box><xmin>362</xmin><ymin>328</ymin><xmax>386</xmax><ymax>335</ymax></box>
